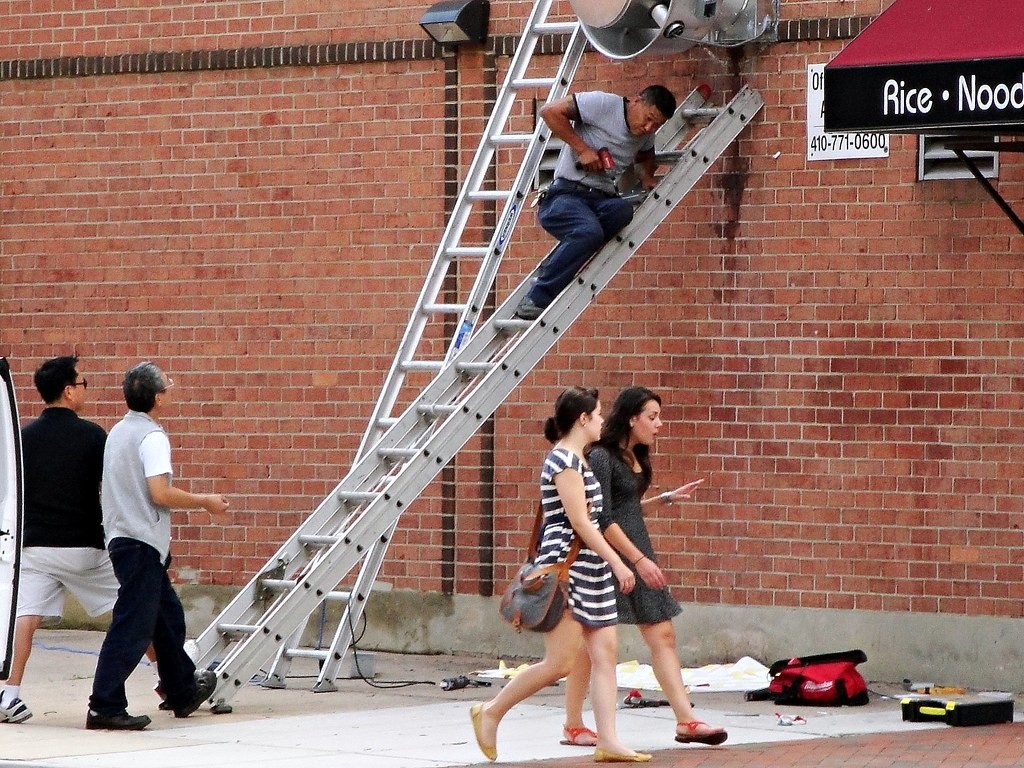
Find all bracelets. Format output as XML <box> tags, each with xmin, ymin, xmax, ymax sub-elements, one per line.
<box><xmin>634</xmin><ymin>556</ymin><xmax>645</xmax><ymax>566</ymax></box>
<box><xmin>661</xmin><ymin>492</ymin><xmax>671</xmax><ymax>503</ymax></box>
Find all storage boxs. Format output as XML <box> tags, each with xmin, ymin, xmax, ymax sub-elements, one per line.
<box><xmin>900</xmin><ymin>693</ymin><xmax>1015</xmax><ymax>727</ymax></box>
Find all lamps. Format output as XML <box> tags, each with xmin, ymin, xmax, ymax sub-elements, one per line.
<box><xmin>419</xmin><ymin>0</ymin><xmax>490</xmax><ymax>46</ymax></box>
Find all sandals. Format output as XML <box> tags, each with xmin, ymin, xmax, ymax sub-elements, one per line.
<box><xmin>559</xmin><ymin>722</ymin><xmax>598</xmax><ymax>745</ymax></box>
<box><xmin>675</xmin><ymin>721</ymin><xmax>728</xmax><ymax>745</ymax></box>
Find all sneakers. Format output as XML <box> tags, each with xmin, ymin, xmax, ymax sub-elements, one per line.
<box><xmin>173</xmin><ymin>670</ymin><xmax>217</xmax><ymax>719</ymax></box>
<box><xmin>513</xmin><ymin>295</ymin><xmax>545</xmax><ymax>321</ymax></box>
<box><xmin>87</xmin><ymin>706</ymin><xmax>151</xmax><ymax>730</ymax></box>
<box><xmin>0</xmin><ymin>690</ymin><xmax>32</xmax><ymax>724</ymax></box>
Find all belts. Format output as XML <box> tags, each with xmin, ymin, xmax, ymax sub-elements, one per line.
<box><xmin>552</xmin><ymin>178</ymin><xmax>604</xmax><ymax>194</ymax></box>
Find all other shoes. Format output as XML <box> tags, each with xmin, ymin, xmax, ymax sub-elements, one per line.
<box><xmin>470</xmin><ymin>703</ymin><xmax>497</xmax><ymax>760</ymax></box>
<box><xmin>594</xmin><ymin>747</ymin><xmax>652</xmax><ymax>762</ymax></box>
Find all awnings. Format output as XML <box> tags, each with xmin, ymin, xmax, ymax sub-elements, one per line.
<box><xmin>824</xmin><ymin>0</ymin><xmax>1024</xmax><ymax>236</ymax></box>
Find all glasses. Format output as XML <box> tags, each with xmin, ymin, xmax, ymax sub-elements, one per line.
<box><xmin>158</xmin><ymin>376</ymin><xmax>174</xmax><ymax>392</ymax></box>
<box><xmin>71</xmin><ymin>378</ymin><xmax>88</xmax><ymax>389</ymax></box>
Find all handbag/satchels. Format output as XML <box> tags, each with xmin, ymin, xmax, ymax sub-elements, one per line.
<box><xmin>499</xmin><ymin>562</ymin><xmax>571</xmax><ymax>631</ymax></box>
<box><xmin>743</xmin><ymin>649</ymin><xmax>869</xmax><ymax>707</ymax></box>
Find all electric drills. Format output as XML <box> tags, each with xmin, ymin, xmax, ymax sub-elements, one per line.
<box><xmin>575</xmin><ymin>144</ymin><xmax>622</xmax><ymax>197</ymax></box>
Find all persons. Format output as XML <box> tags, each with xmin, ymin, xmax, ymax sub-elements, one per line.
<box><xmin>85</xmin><ymin>362</ymin><xmax>230</xmax><ymax>730</ymax></box>
<box><xmin>514</xmin><ymin>84</ymin><xmax>677</xmax><ymax>321</ymax></box>
<box><xmin>559</xmin><ymin>385</ymin><xmax>728</xmax><ymax>746</ymax></box>
<box><xmin>0</xmin><ymin>356</ymin><xmax>201</xmax><ymax>724</ymax></box>
<box><xmin>468</xmin><ymin>386</ymin><xmax>653</xmax><ymax>762</ymax></box>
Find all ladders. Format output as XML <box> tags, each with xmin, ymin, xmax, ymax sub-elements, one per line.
<box><xmin>162</xmin><ymin>0</ymin><xmax>765</xmax><ymax>712</ymax></box>
